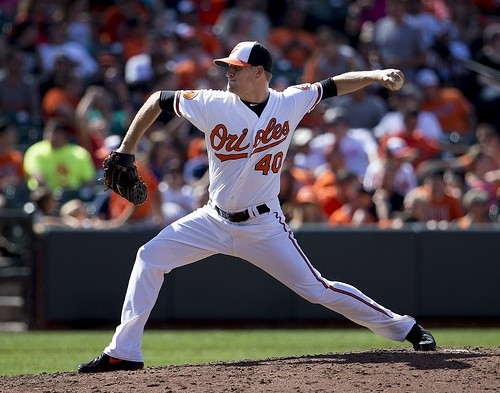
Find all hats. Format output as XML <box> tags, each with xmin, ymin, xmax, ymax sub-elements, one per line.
<box><xmin>214</xmin><ymin>40</ymin><xmax>272</xmax><ymax>74</ymax></box>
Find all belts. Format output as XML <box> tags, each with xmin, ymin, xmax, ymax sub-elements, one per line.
<box><xmin>215</xmin><ymin>204</ymin><xmax>270</xmax><ymax>223</ymax></box>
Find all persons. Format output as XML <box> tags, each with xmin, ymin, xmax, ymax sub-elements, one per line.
<box><xmin>78</xmin><ymin>41</ymin><xmax>436</xmax><ymax>373</ymax></box>
<box><xmin>0</xmin><ymin>0</ymin><xmax>500</xmax><ymax>232</ymax></box>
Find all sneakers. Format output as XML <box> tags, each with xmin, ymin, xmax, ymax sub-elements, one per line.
<box><xmin>404</xmin><ymin>324</ymin><xmax>436</xmax><ymax>351</ymax></box>
<box><xmin>78</xmin><ymin>353</ymin><xmax>143</xmax><ymax>374</ymax></box>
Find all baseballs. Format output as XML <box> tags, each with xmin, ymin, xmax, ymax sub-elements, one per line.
<box><xmin>387</xmin><ymin>74</ymin><xmax>404</xmax><ymax>91</ymax></box>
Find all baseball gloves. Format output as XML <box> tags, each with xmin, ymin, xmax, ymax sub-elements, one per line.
<box><xmin>103</xmin><ymin>151</ymin><xmax>148</xmax><ymax>206</ymax></box>
